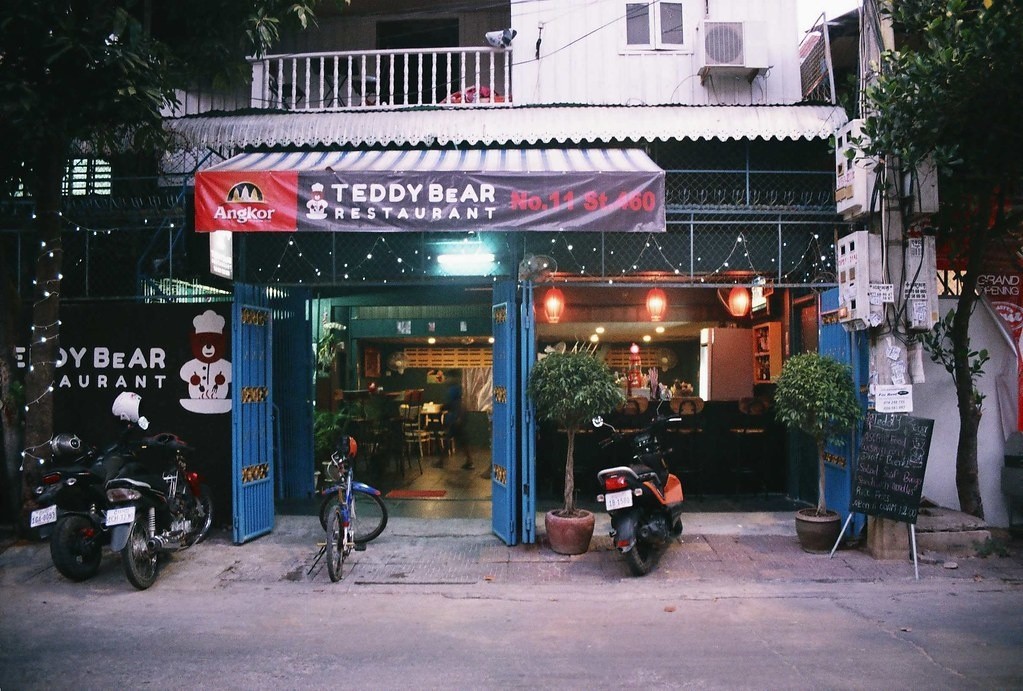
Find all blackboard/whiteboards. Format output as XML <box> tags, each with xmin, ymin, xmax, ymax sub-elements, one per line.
<box><xmin>850</xmin><ymin>410</ymin><xmax>935</xmax><ymax>524</ymax></box>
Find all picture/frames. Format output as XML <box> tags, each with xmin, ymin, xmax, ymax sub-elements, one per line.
<box><xmin>365</xmin><ymin>348</ymin><xmax>381</xmax><ymax>378</ymax></box>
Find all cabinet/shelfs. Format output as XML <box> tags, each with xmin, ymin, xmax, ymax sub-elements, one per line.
<box><xmin>751</xmin><ymin>322</ymin><xmax>783</xmax><ymax>385</ymax></box>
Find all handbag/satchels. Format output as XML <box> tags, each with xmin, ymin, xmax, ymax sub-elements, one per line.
<box><xmin>452</xmin><ymin>414</ymin><xmax>464</xmax><ymax>429</ymax></box>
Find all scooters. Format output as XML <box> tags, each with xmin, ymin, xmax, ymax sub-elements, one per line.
<box><xmin>589</xmin><ymin>387</ymin><xmax>685</xmax><ymax>577</ymax></box>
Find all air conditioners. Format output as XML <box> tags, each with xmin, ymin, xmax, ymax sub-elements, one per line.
<box><xmin>693</xmin><ymin>17</ymin><xmax>774</xmax><ymax>84</ymax></box>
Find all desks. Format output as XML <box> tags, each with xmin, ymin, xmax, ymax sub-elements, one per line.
<box><xmin>557</xmin><ymin>427</ymin><xmax>771</xmax><ymax>502</ymax></box>
<box><xmin>420</xmin><ymin>402</ymin><xmax>443</xmax><ymax>453</ymax></box>
<box><xmin>310</xmin><ymin>58</ymin><xmax>358</xmax><ymax>105</ymax></box>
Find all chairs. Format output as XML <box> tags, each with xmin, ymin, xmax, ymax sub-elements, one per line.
<box><xmin>270</xmin><ymin>62</ymin><xmax>306</xmax><ymax>108</ymax></box>
<box><xmin>352</xmin><ymin>47</ymin><xmax>390</xmax><ymax>105</ymax></box>
<box><xmin>344</xmin><ymin>389</ymin><xmax>456</xmax><ymax>478</ymax></box>
<box><xmin>539</xmin><ymin>396</ymin><xmax>768</xmax><ymax>503</ymax></box>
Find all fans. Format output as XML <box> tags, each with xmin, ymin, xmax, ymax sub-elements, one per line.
<box><xmin>387</xmin><ymin>351</ymin><xmax>411</xmax><ymax>375</ymax></box>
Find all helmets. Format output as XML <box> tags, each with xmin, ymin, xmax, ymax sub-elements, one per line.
<box><xmin>345</xmin><ymin>436</ymin><xmax>358</xmax><ymax>460</ymax></box>
<box><xmin>111</xmin><ymin>391</ymin><xmax>142</xmax><ymax>423</ymax></box>
<box><xmin>51</xmin><ymin>433</ymin><xmax>88</xmax><ymax>464</ymax></box>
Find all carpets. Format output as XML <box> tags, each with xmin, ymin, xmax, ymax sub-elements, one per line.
<box><xmin>385</xmin><ymin>489</ymin><xmax>446</xmax><ymax>497</ymax></box>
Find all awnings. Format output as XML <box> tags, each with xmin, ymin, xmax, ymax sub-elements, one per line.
<box><xmin>193</xmin><ymin>149</ymin><xmax>668</xmax><ymax>234</ymax></box>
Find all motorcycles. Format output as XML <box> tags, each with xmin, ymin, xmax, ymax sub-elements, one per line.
<box><xmin>28</xmin><ymin>414</ymin><xmax>216</xmax><ymax>591</ymax></box>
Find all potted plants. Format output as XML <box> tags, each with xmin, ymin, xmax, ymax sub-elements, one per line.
<box><xmin>774</xmin><ymin>349</ymin><xmax>865</xmax><ymax>555</ymax></box>
<box><xmin>530</xmin><ymin>352</ymin><xmax>628</xmax><ymax>556</ymax></box>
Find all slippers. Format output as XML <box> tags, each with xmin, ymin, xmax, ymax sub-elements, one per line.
<box><xmin>461</xmin><ymin>463</ymin><xmax>475</xmax><ymax>469</ymax></box>
<box><xmin>432</xmin><ymin>461</ymin><xmax>444</xmax><ymax>469</ymax></box>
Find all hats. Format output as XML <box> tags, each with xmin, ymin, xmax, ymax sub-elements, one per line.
<box><xmin>444</xmin><ymin>369</ymin><xmax>458</xmax><ymax>377</ymax></box>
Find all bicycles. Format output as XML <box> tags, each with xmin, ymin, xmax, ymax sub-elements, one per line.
<box><xmin>306</xmin><ymin>432</ymin><xmax>390</xmax><ymax>583</ymax></box>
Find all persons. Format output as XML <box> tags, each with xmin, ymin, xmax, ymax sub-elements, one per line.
<box><xmin>432</xmin><ymin>370</ymin><xmax>476</xmax><ymax>471</ymax></box>
